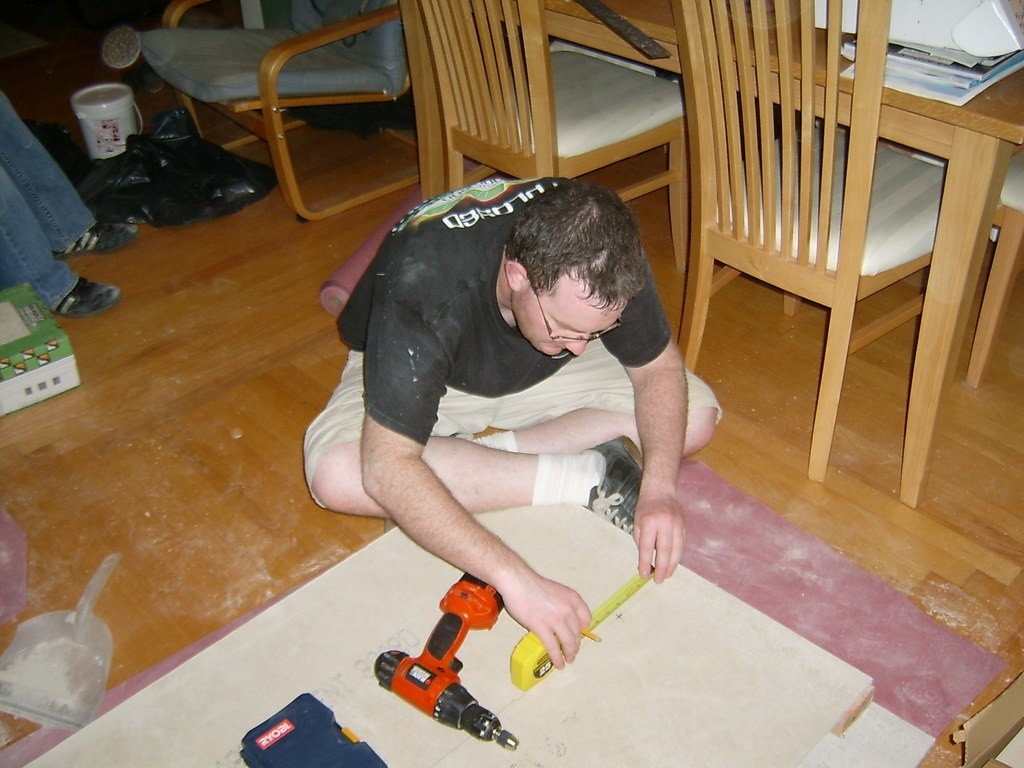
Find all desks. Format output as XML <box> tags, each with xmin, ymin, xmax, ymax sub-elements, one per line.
<box><xmin>402</xmin><ymin>0</ymin><xmax>1024</xmax><ymax>512</ymax></box>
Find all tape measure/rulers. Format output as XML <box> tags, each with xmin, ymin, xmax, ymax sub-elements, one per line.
<box><xmin>511</xmin><ymin>568</ymin><xmax>659</xmax><ymax>690</ymax></box>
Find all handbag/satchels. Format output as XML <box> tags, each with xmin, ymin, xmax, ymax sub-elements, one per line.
<box><xmin>83</xmin><ymin>106</ymin><xmax>277</xmax><ymax>228</ymax></box>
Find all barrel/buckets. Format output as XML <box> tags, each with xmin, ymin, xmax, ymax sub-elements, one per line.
<box><xmin>70</xmin><ymin>84</ymin><xmax>143</xmax><ymax>160</ymax></box>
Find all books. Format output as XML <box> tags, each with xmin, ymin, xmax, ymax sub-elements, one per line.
<box><xmin>841</xmin><ymin>37</ymin><xmax>1024</xmax><ymax>107</ymax></box>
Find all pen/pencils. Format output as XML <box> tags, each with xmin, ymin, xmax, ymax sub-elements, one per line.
<box><xmin>579</xmin><ymin>627</ymin><xmax>602</xmax><ymax>644</ymax></box>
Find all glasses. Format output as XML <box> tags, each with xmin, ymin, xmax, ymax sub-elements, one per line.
<box><xmin>534</xmin><ymin>291</ymin><xmax>623</xmax><ymax>343</ymax></box>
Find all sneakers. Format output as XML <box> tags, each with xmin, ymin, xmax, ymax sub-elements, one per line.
<box><xmin>581</xmin><ymin>434</ymin><xmax>645</xmax><ymax>535</ymax></box>
<box><xmin>56</xmin><ymin>276</ymin><xmax>122</xmax><ymax>317</ymax></box>
<box><xmin>55</xmin><ymin>220</ymin><xmax>138</xmax><ymax>256</ymax></box>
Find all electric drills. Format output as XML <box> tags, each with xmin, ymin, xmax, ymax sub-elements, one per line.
<box><xmin>372</xmin><ymin>573</ymin><xmax>520</xmax><ymax>751</ymax></box>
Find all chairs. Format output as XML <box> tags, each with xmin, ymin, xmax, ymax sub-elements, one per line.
<box><xmin>673</xmin><ymin>0</ymin><xmax>945</xmax><ymax>486</ymax></box>
<box><xmin>139</xmin><ymin>0</ymin><xmax>422</xmax><ymax>221</ymax></box>
<box><xmin>418</xmin><ymin>0</ymin><xmax>689</xmax><ymax>273</ymax></box>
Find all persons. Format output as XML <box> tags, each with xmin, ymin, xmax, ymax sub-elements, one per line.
<box><xmin>304</xmin><ymin>177</ymin><xmax>722</xmax><ymax>669</ymax></box>
<box><xmin>0</xmin><ymin>92</ymin><xmax>137</xmax><ymax>318</ymax></box>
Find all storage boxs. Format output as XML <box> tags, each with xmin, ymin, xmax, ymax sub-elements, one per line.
<box><xmin>0</xmin><ymin>283</ymin><xmax>82</xmax><ymax>417</ymax></box>
